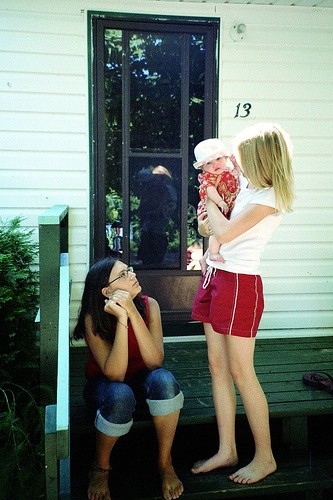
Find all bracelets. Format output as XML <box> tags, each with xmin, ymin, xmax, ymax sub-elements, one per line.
<box><xmin>118</xmin><ymin>319</ymin><xmax>129</xmax><ymax>330</ymax></box>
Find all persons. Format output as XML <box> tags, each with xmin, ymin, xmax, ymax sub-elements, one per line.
<box><xmin>188</xmin><ymin>127</ymin><xmax>297</xmax><ymax>485</ymax></box>
<box><xmin>70</xmin><ymin>257</ymin><xmax>187</xmax><ymax>500</ymax></box>
<box><xmin>136</xmin><ymin>167</ymin><xmax>178</xmax><ymax>269</ymax></box>
<box><xmin>190</xmin><ymin>139</ymin><xmax>242</xmax><ymax>277</ymax></box>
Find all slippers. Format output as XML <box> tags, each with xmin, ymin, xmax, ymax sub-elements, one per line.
<box><xmin>302</xmin><ymin>370</ymin><xmax>333</xmax><ymax>396</ymax></box>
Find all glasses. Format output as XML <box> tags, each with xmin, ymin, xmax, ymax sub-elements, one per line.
<box><xmin>104</xmin><ymin>265</ymin><xmax>134</xmax><ymax>288</ymax></box>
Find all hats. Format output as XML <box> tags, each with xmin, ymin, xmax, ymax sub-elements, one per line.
<box><xmin>192</xmin><ymin>137</ymin><xmax>231</xmax><ymax>169</ymax></box>
